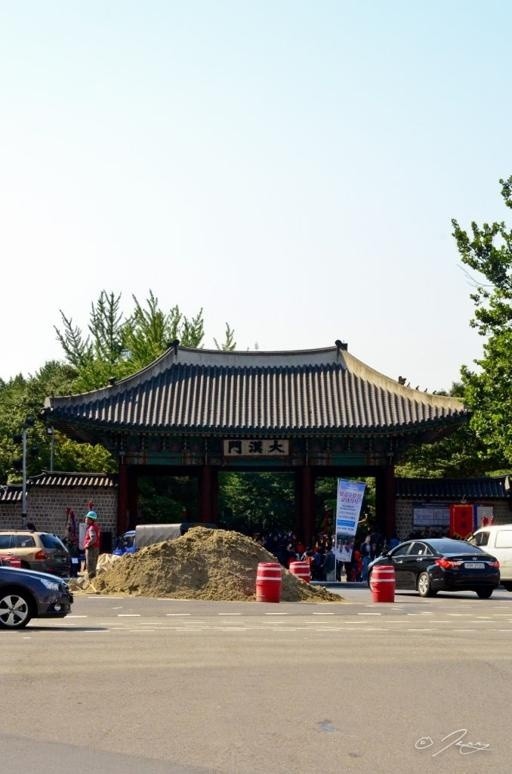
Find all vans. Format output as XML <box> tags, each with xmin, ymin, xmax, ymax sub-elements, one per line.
<box><xmin>467</xmin><ymin>523</ymin><xmax>512</xmax><ymax>592</ymax></box>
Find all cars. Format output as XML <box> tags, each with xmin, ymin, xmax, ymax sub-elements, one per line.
<box><xmin>367</xmin><ymin>537</ymin><xmax>500</xmax><ymax>598</ymax></box>
<box><xmin>0</xmin><ymin>529</ymin><xmax>74</xmax><ymax>630</ymax></box>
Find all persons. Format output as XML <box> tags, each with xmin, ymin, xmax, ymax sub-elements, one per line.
<box><xmin>241</xmin><ymin>525</ymin><xmax>449</xmax><ymax>583</ymax></box>
<box><xmin>26</xmin><ymin>523</ymin><xmax>37</xmax><ymax>531</ymax></box>
<box><xmin>82</xmin><ymin>510</ymin><xmax>102</xmax><ymax>579</ymax></box>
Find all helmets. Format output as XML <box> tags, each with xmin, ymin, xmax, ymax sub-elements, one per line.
<box><xmin>85</xmin><ymin>511</ymin><xmax>98</xmax><ymax>520</ymax></box>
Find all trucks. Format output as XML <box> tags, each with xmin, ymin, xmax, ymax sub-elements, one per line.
<box><xmin>111</xmin><ymin>523</ymin><xmax>183</xmax><ymax>556</ymax></box>
